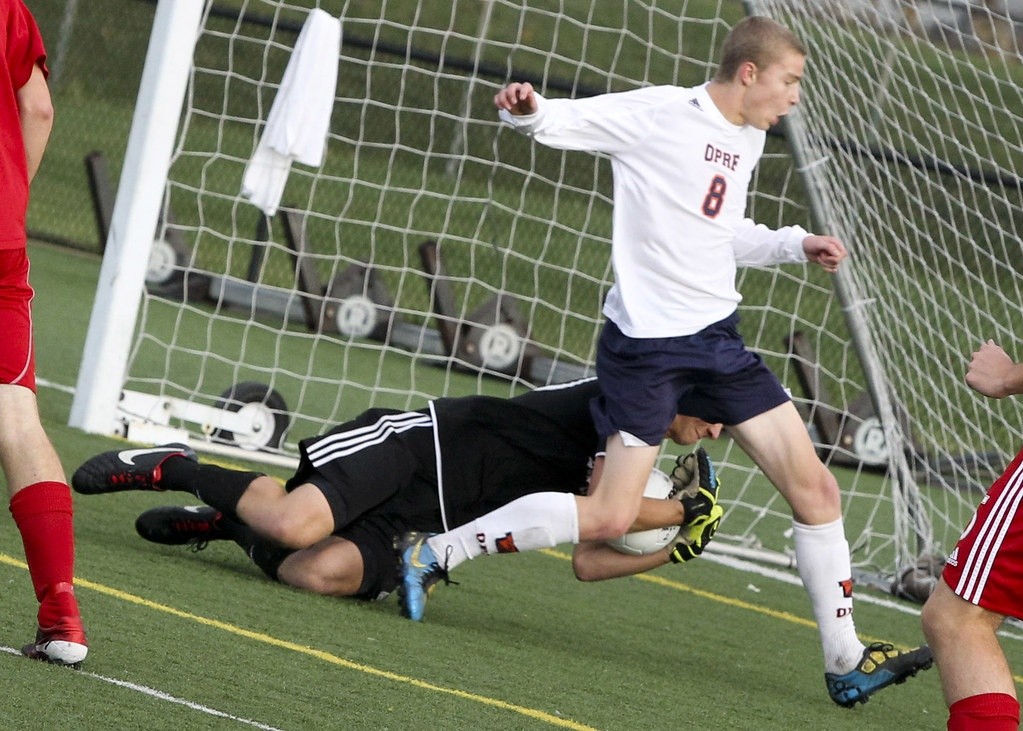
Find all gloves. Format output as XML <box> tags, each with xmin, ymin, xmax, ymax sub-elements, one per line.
<box><xmin>667</xmin><ymin>504</ymin><xmax>723</xmax><ymax>565</ymax></box>
<box><xmin>670</xmin><ymin>447</ymin><xmax>720</xmax><ymax>527</ymax></box>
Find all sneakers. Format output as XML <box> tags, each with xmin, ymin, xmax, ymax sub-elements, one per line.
<box><xmin>392</xmin><ymin>532</ymin><xmax>460</xmax><ymax>622</ymax></box>
<box><xmin>824</xmin><ymin>642</ymin><xmax>933</xmax><ymax>709</ymax></box>
<box><xmin>20</xmin><ymin>615</ymin><xmax>89</xmax><ymax>671</ymax></box>
<box><xmin>71</xmin><ymin>442</ymin><xmax>199</xmax><ymax>494</ymax></box>
<box><xmin>135</xmin><ymin>506</ymin><xmax>237</xmax><ymax>553</ymax></box>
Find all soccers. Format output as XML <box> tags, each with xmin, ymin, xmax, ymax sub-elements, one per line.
<box><xmin>599</xmin><ymin>467</ymin><xmax>682</xmax><ymax>556</ymax></box>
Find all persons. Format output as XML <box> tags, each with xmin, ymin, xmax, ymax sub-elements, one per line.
<box><xmin>72</xmin><ymin>375</ymin><xmax>723</xmax><ymax>596</ymax></box>
<box><xmin>394</xmin><ymin>16</ymin><xmax>938</xmax><ymax>708</ymax></box>
<box><xmin>923</xmin><ymin>339</ymin><xmax>1023</xmax><ymax>731</ymax></box>
<box><xmin>0</xmin><ymin>0</ymin><xmax>89</xmax><ymax>669</ymax></box>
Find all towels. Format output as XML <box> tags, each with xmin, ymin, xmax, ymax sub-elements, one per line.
<box><xmin>240</xmin><ymin>7</ymin><xmax>342</xmax><ymax>217</ymax></box>
